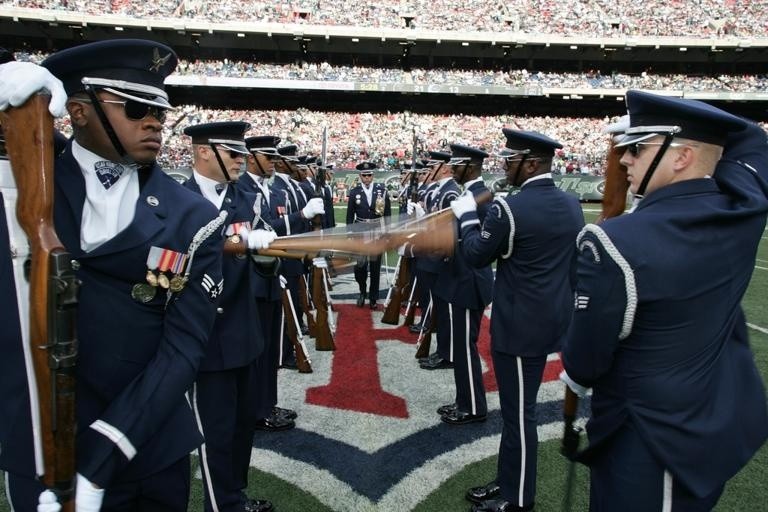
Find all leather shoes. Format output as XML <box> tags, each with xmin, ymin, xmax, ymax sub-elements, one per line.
<box><xmin>442</xmin><ymin>411</ymin><xmax>488</xmax><ymax>423</ymax></box>
<box><xmin>256</xmin><ymin>416</ymin><xmax>296</xmax><ymax>432</ymax></box>
<box><xmin>357</xmin><ymin>295</ymin><xmax>380</xmax><ymax>309</ymax></box>
<box><xmin>465</xmin><ymin>480</ymin><xmax>502</xmax><ymax>501</ymax></box>
<box><xmin>437</xmin><ymin>404</ymin><xmax>461</xmax><ymax>413</ymax></box>
<box><xmin>401</xmin><ymin>297</ymin><xmax>451</xmax><ymax>370</ymax></box>
<box><xmin>271</xmin><ymin>407</ymin><xmax>299</xmax><ymax>419</ymax></box>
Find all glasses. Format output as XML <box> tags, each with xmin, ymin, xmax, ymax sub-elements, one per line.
<box><xmin>217</xmin><ymin>145</ymin><xmax>244</xmax><ymax>159</ymax></box>
<box><xmin>83</xmin><ymin>96</ymin><xmax>167</xmax><ymax>124</ymax></box>
<box><xmin>628</xmin><ymin>141</ymin><xmax>683</xmax><ymax>157</ymax></box>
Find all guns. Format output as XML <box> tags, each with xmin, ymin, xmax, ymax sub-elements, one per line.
<box><xmin>560</xmin><ymin>129</ymin><xmax>631</xmax><ymax>509</ymax></box>
<box><xmin>223</xmin><ymin>208</ymin><xmax>458</xmax><ymax>258</ymax></box>
<box><xmin>0</xmin><ymin>92</ymin><xmax>77</xmax><ymax>511</ymax></box>
<box><xmin>381</xmin><ymin>131</ymin><xmax>436</xmax><ymax>359</ymax></box>
<box><xmin>274</xmin><ymin>124</ymin><xmax>335</xmax><ymax>374</ymax></box>
<box><xmin>324</xmin><ymin>174</ymin><xmax>508</xmax><ymax>273</ymax></box>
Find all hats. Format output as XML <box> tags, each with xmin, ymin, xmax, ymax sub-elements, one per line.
<box><xmin>500</xmin><ymin>126</ymin><xmax>563</xmax><ymax>160</ymax></box>
<box><xmin>298</xmin><ymin>156</ymin><xmax>334</xmax><ymax>173</ymax></box>
<box><xmin>445</xmin><ymin>143</ymin><xmax>488</xmax><ymax>167</ymax></box>
<box><xmin>400</xmin><ymin>151</ymin><xmax>449</xmax><ymax>172</ymax></box>
<box><xmin>614</xmin><ymin>89</ymin><xmax>753</xmax><ymax>150</ymax></box>
<box><xmin>40</xmin><ymin>38</ymin><xmax>177</xmax><ymax>112</ymax></box>
<box><xmin>356</xmin><ymin>161</ymin><xmax>376</xmax><ymax>175</ymax></box>
<box><xmin>245</xmin><ymin>135</ymin><xmax>281</xmax><ymax>158</ymax></box>
<box><xmin>275</xmin><ymin>144</ymin><xmax>298</xmax><ymax>162</ymax></box>
<box><xmin>184</xmin><ymin>122</ymin><xmax>252</xmax><ymax>155</ymax></box>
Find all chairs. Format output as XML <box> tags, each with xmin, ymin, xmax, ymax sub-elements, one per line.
<box><xmin>0</xmin><ymin>0</ymin><xmax>768</xmax><ymax>183</ymax></box>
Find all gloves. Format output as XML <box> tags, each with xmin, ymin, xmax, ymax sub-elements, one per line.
<box><xmin>33</xmin><ymin>473</ymin><xmax>104</xmax><ymax>512</ymax></box>
<box><xmin>0</xmin><ymin>61</ymin><xmax>68</xmax><ymax>117</ymax></box>
<box><xmin>407</xmin><ymin>199</ymin><xmax>425</xmax><ymax>220</ymax></box>
<box><xmin>302</xmin><ymin>197</ymin><xmax>325</xmax><ymax>219</ymax></box>
<box><xmin>451</xmin><ymin>190</ymin><xmax>477</xmax><ymax>218</ymax></box>
<box><xmin>247</xmin><ymin>229</ymin><xmax>279</xmax><ymax>250</ymax></box>
